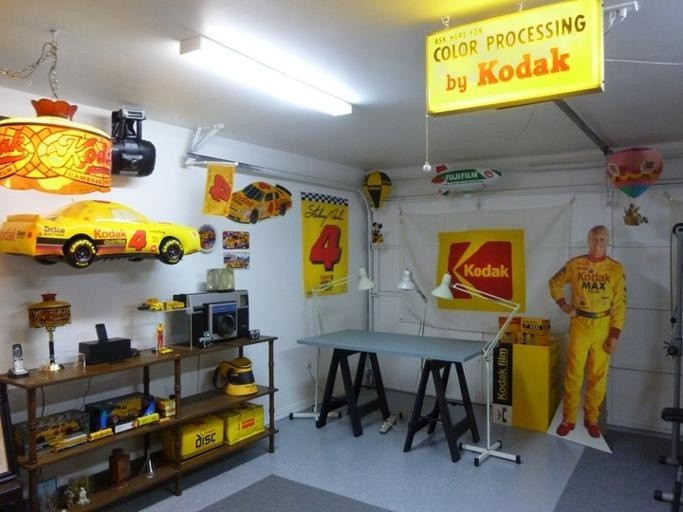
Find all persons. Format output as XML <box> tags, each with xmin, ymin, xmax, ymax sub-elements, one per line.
<box><xmin>549</xmin><ymin>225</ymin><xmax>628</xmax><ymax>437</ymax></box>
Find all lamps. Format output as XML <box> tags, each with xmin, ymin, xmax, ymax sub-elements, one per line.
<box><xmin>290</xmin><ymin>267</ymin><xmax>521</xmax><ymax>466</ymax></box>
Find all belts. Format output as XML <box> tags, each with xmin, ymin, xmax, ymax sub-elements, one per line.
<box><xmin>574</xmin><ymin>309</ymin><xmax>610</xmax><ymax>318</ymax></box>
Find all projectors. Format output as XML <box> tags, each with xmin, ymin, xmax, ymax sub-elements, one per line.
<box><xmin>112</xmin><ymin>140</ymin><xmax>155</xmax><ymax>176</ymax></box>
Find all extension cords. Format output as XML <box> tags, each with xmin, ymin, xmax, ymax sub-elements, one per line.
<box><xmin>379</xmin><ymin>414</ymin><xmax>397</xmax><ymax>434</ymax></box>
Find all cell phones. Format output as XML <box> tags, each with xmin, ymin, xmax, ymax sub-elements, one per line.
<box><xmin>95</xmin><ymin>324</ymin><xmax>107</xmax><ymax>341</ymax></box>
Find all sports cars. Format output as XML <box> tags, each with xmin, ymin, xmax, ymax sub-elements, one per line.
<box><xmin>223</xmin><ymin>180</ymin><xmax>293</xmax><ymax>225</ymax></box>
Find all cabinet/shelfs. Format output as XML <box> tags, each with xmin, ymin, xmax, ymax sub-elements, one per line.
<box><xmin>0</xmin><ymin>334</ymin><xmax>279</xmax><ymax>510</ymax></box>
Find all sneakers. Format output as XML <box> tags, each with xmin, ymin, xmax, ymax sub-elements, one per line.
<box><xmin>557</xmin><ymin>421</ymin><xmax>575</xmax><ymax>435</ymax></box>
<box><xmin>585</xmin><ymin>420</ymin><xmax>600</xmax><ymax>437</ymax></box>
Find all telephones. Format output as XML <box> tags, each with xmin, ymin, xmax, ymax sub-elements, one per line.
<box><xmin>9</xmin><ymin>344</ymin><xmax>30</xmax><ymax>378</ymax></box>
